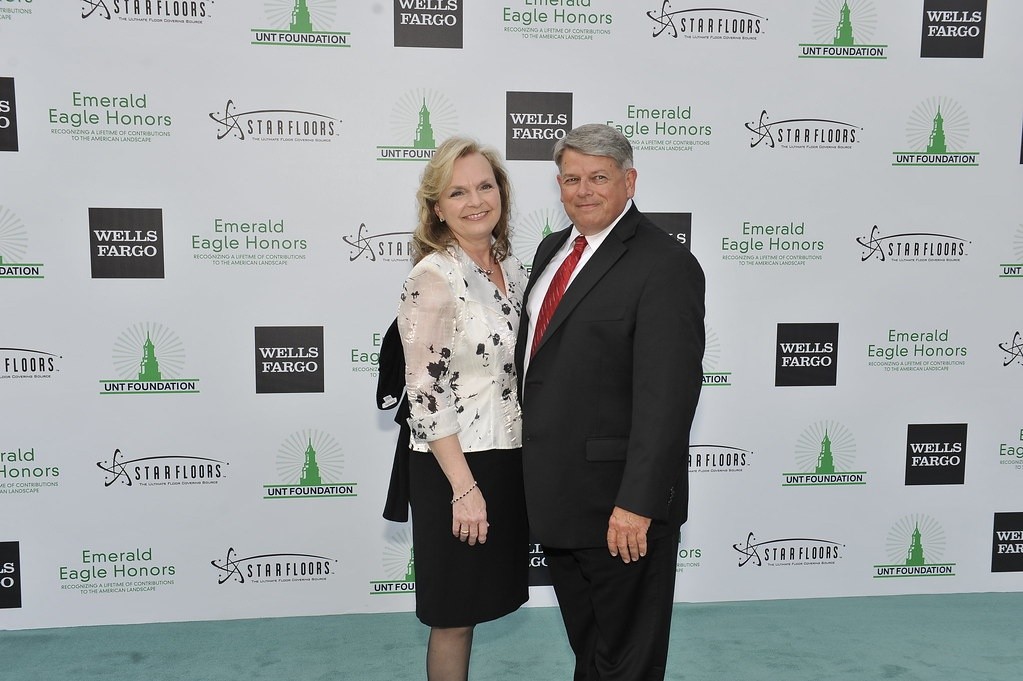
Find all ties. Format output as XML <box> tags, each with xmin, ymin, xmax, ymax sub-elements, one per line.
<box><xmin>530</xmin><ymin>236</ymin><xmax>588</xmax><ymax>357</ymax></box>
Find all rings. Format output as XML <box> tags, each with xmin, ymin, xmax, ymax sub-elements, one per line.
<box><xmin>459</xmin><ymin>528</ymin><xmax>470</xmax><ymax>534</ymax></box>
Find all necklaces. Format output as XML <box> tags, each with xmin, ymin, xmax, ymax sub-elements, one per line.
<box><xmin>466</xmin><ymin>255</ymin><xmax>497</xmax><ymax>276</ymax></box>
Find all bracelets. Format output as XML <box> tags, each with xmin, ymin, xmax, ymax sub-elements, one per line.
<box><xmin>449</xmin><ymin>480</ymin><xmax>479</xmax><ymax>504</ymax></box>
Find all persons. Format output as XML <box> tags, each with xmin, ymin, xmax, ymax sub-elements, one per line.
<box><xmin>397</xmin><ymin>133</ymin><xmax>540</xmax><ymax>680</ymax></box>
<box><xmin>512</xmin><ymin>123</ymin><xmax>709</xmax><ymax>681</ymax></box>
